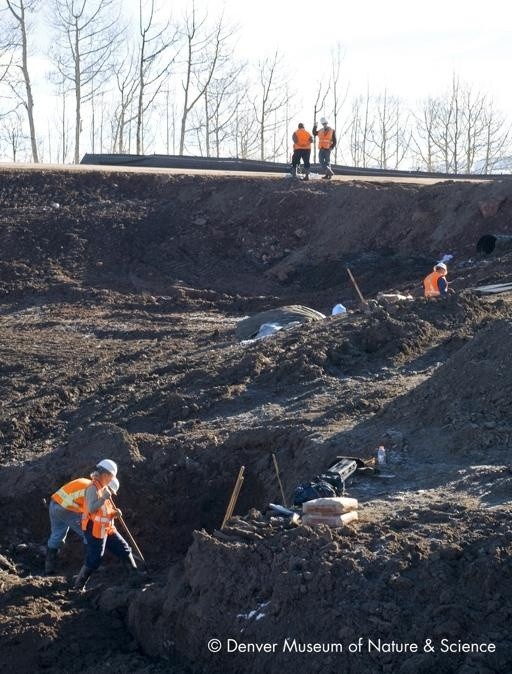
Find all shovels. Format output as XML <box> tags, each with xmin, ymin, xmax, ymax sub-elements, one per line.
<box><xmin>347</xmin><ymin>268</ymin><xmax>369</xmax><ymax>311</ymax></box>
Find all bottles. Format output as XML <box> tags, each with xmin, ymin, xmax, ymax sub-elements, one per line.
<box><xmin>378</xmin><ymin>446</ymin><xmax>386</xmax><ymax>464</ymax></box>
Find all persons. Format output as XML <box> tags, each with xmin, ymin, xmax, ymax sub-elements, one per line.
<box><xmin>74</xmin><ymin>459</ymin><xmax>152</xmax><ymax>592</ymax></box>
<box><xmin>313</xmin><ymin>118</ymin><xmax>337</xmax><ymax>179</ymax></box>
<box><xmin>422</xmin><ymin>262</ymin><xmax>449</xmax><ymax>299</ymax></box>
<box><xmin>44</xmin><ymin>473</ymin><xmax>120</xmax><ymax>577</ymax></box>
<box><xmin>292</xmin><ymin>123</ymin><xmax>313</xmax><ymax>181</ymax></box>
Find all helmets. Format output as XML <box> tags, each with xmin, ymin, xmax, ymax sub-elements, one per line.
<box><xmin>108</xmin><ymin>479</ymin><xmax>120</xmax><ymax>496</ymax></box>
<box><xmin>320</xmin><ymin>118</ymin><xmax>328</xmax><ymax>124</ymax></box>
<box><xmin>434</xmin><ymin>263</ymin><xmax>448</xmax><ymax>275</ymax></box>
<box><xmin>97</xmin><ymin>458</ymin><xmax>118</xmax><ymax>479</ymax></box>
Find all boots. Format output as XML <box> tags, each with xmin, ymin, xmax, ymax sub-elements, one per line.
<box><xmin>125</xmin><ymin>554</ymin><xmax>154</xmax><ymax>586</ymax></box>
<box><xmin>322</xmin><ymin>166</ymin><xmax>334</xmax><ymax>180</ymax></box>
<box><xmin>293</xmin><ymin>167</ymin><xmax>309</xmax><ymax>181</ymax></box>
<box><xmin>75</xmin><ymin>566</ymin><xmax>93</xmax><ymax>591</ymax></box>
<box><xmin>45</xmin><ymin>548</ymin><xmax>61</xmax><ymax>576</ymax></box>
